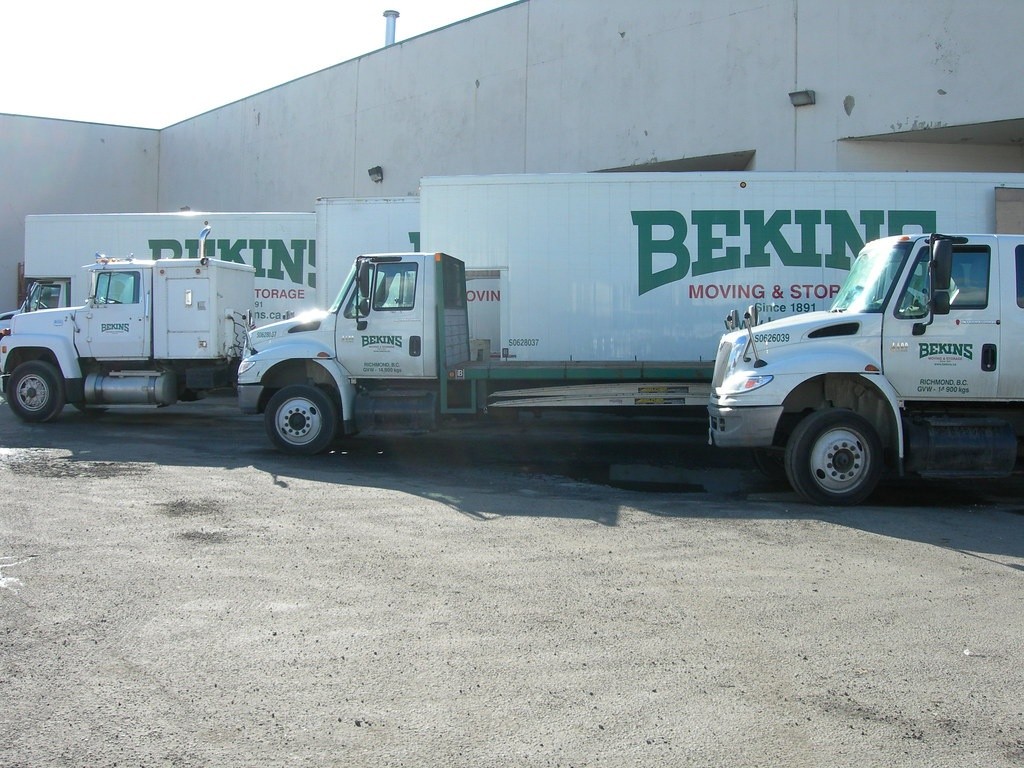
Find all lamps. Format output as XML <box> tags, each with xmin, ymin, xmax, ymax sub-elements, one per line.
<box><xmin>789</xmin><ymin>89</ymin><xmax>815</xmax><ymax>107</ymax></box>
<box><xmin>368</xmin><ymin>166</ymin><xmax>383</xmax><ymax>182</ymax></box>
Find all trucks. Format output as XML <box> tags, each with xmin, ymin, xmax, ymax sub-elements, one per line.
<box><xmin>704</xmin><ymin>231</ymin><xmax>1024</xmax><ymax>505</ymax></box>
<box><xmin>0</xmin><ymin>196</ymin><xmax>427</xmax><ymax>423</ymax></box>
<box><xmin>228</xmin><ymin>171</ymin><xmax>1022</xmax><ymax>452</ymax></box>
<box><xmin>0</xmin><ymin>213</ymin><xmax>317</xmax><ymax>324</ymax></box>
